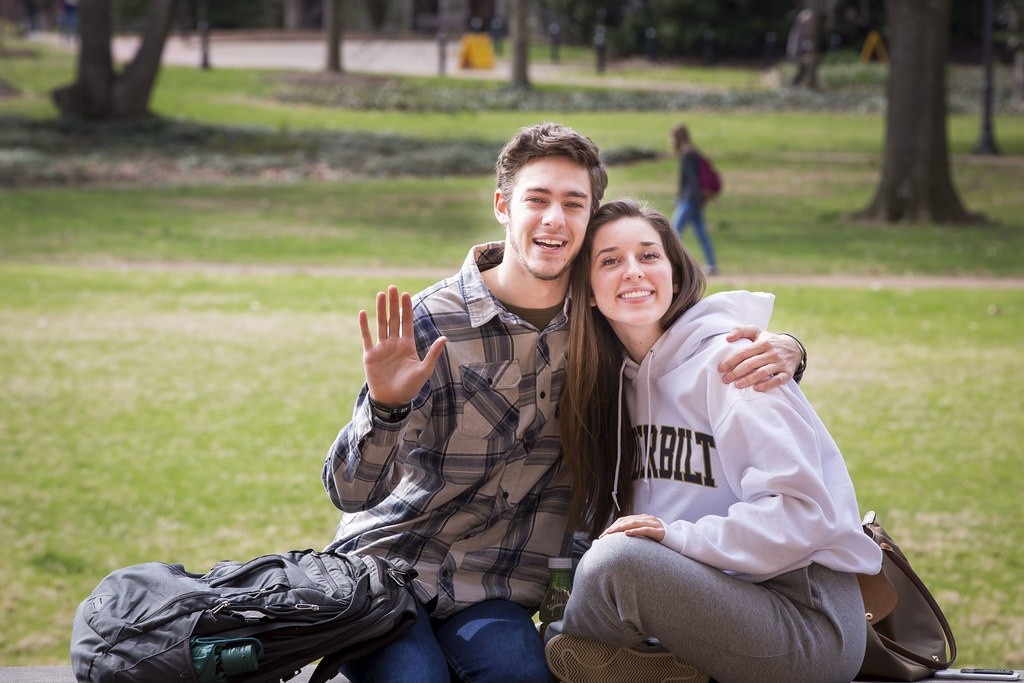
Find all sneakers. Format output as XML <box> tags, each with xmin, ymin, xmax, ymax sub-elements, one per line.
<box><xmin>546</xmin><ymin>633</ymin><xmax>712</xmax><ymax>683</ymax></box>
<box><xmin>701</xmin><ymin>265</ymin><xmax>720</xmax><ymax>277</ymax></box>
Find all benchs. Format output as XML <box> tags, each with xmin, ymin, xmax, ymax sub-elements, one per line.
<box><xmin>0</xmin><ymin>665</ymin><xmax>1023</xmax><ymax>682</ymax></box>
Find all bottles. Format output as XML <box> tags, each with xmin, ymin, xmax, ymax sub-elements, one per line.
<box><xmin>540</xmin><ymin>557</ymin><xmax>575</xmax><ymax>640</ymax></box>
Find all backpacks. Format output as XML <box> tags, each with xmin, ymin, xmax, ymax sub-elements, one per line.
<box><xmin>70</xmin><ymin>546</ymin><xmax>418</xmax><ymax>683</ymax></box>
<box><xmin>695</xmin><ymin>154</ymin><xmax>720</xmax><ymax>198</ymax></box>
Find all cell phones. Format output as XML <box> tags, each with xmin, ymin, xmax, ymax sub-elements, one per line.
<box><xmin>934</xmin><ymin>669</ymin><xmax>1020</xmax><ymax>681</ymax></box>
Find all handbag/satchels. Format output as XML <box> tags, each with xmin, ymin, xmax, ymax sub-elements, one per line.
<box><xmin>855</xmin><ymin>511</ymin><xmax>957</xmax><ymax>682</ymax></box>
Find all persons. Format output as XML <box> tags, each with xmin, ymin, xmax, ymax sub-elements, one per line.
<box><xmin>788</xmin><ymin>10</ymin><xmax>820</xmax><ymax>96</ymax></box>
<box><xmin>322</xmin><ymin>123</ymin><xmax>809</xmax><ymax>682</ymax></box>
<box><xmin>664</xmin><ymin>123</ymin><xmax>722</xmax><ymax>282</ymax></box>
<box><xmin>540</xmin><ymin>194</ymin><xmax>887</xmax><ymax>683</ymax></box>
